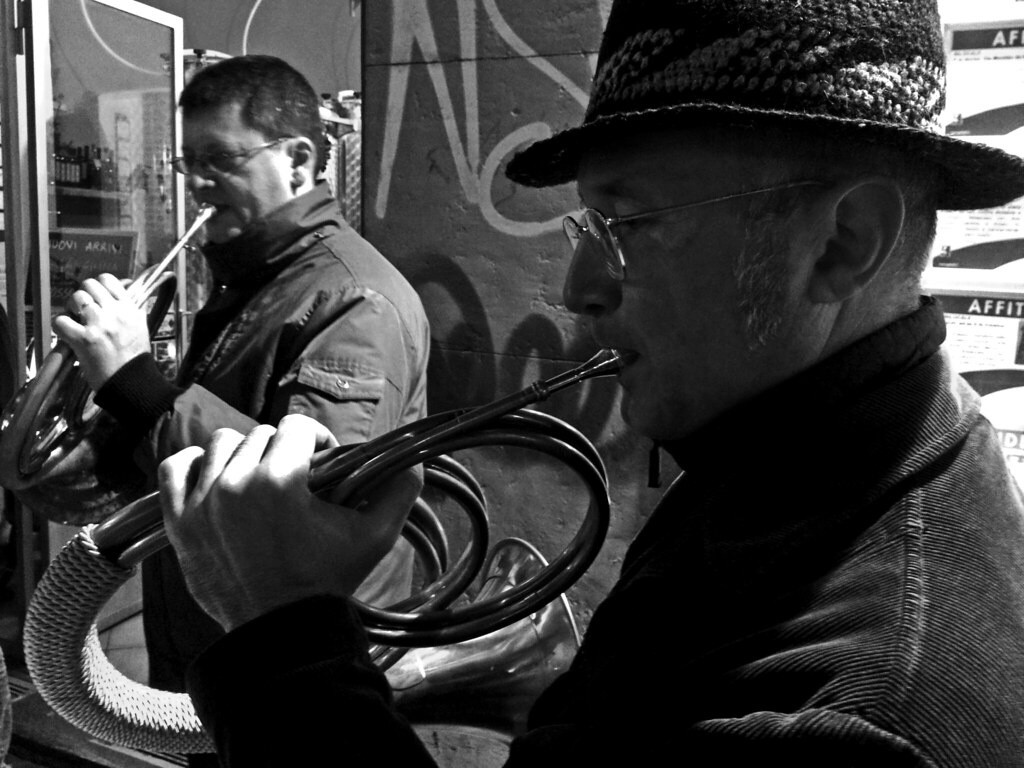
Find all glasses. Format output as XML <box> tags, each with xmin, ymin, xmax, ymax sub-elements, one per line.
<box><xmin>169</xmin><ymin>136</ymin><xmax>290</xmax><ymax>175</ymax></box>
<box><xmin>562</xmin><ymin>179</ymin><xmax>839</xmax><ymax>280</ymax></box>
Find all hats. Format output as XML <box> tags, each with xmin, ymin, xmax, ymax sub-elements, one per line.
<box><xmin>502</xmin><ymin>2</ymin><xmax>1022</xmax><ymax>212</ymax></box>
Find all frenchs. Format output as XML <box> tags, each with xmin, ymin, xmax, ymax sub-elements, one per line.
<box><xmin>23</xmin><ymin>335</ymin><xmax>633</xmax><ymax>755</ymax></box>
<box><xmin>0</xmin><ymin>199</ymin><xmax>219</xmax><ymax>499</ymax></box>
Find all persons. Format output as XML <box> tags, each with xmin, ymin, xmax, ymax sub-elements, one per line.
<box><xmin>51</xmin><ymin>54</ymin><xmax>433</xmax><ymax>767</ymax></box>
<box><xmin>156</xmin><ymin>2</ymin><xmax>1024</xmax><ymax>768</ymax></box>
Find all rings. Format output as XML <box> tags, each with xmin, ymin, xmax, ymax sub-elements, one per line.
<box><xmin>74</xmin><ymin>298</ymin><xmax>95</xmax><ymax>317</ymax></box>
